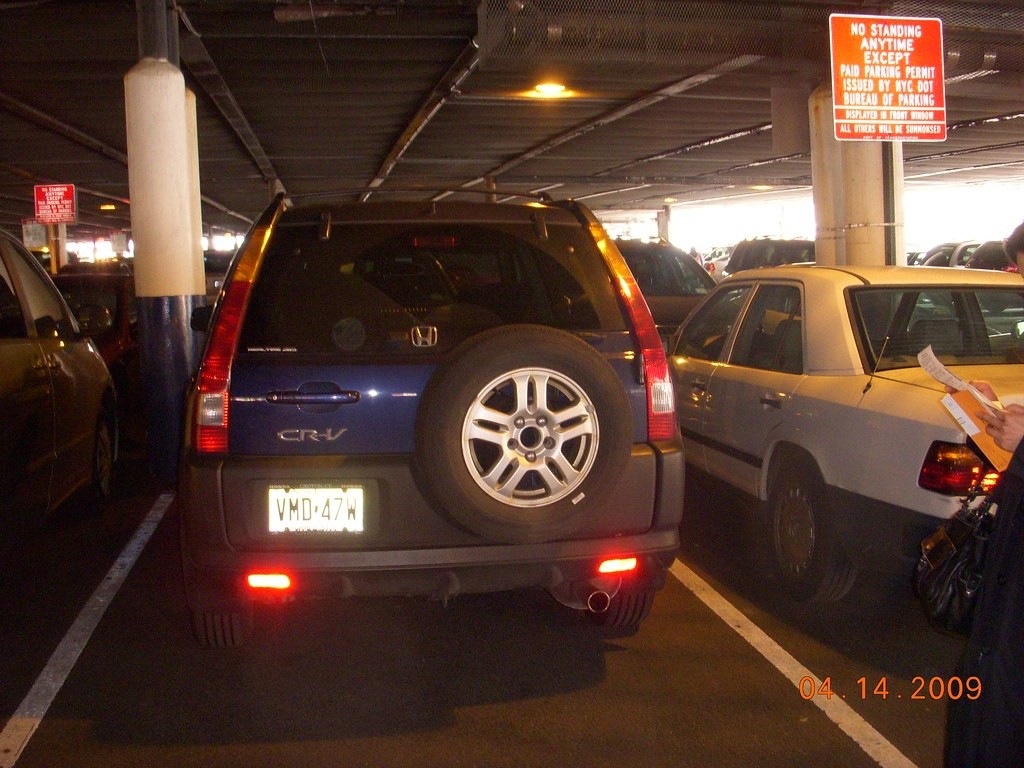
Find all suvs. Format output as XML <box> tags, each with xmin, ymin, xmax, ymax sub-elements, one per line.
<box><xmin>185</xmin><ymin>189</ymin><xmax>688</xmax><ymax>651</ymax></box>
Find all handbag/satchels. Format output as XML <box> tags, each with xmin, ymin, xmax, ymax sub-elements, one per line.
<box><xmin>916</xmin><ymin>459</ymin><xmax>997</xmax><ymax>640</ymax></box>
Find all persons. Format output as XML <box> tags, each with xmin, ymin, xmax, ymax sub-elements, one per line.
<box><xmin>689</xmin><ymin>246</ymin><xmax>705</xmax><ymax>266</ymax></box>
<box><xmin>941</xmin><ymin>222</ymin><xmax>1024</xmax><ymax>768</ymax></box>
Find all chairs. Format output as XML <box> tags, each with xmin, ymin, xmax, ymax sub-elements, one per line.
<box><xmin>908</xmin><ymin>317</ymin><xmax>963</xmax><ymax>357</ymax></box>
<box><xmin>767</xmin><ymin>319</ymin><xmax>802</xmax><ymax>375</ymax></box>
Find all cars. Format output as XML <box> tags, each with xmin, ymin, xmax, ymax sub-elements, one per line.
<box><xmin>610</xmin><ymin>239</ymin><xmax>719</xmax><ymax>343</ymax></box>
<box><xmin>663</xmin><ymin>235</ymin><xmax>1024</xmax><ymax>607</ymax></box>
<box><xmin>0</xmin><ymin>226</ymin><xmax>235</xmax><ymax>551</ymax></box>
